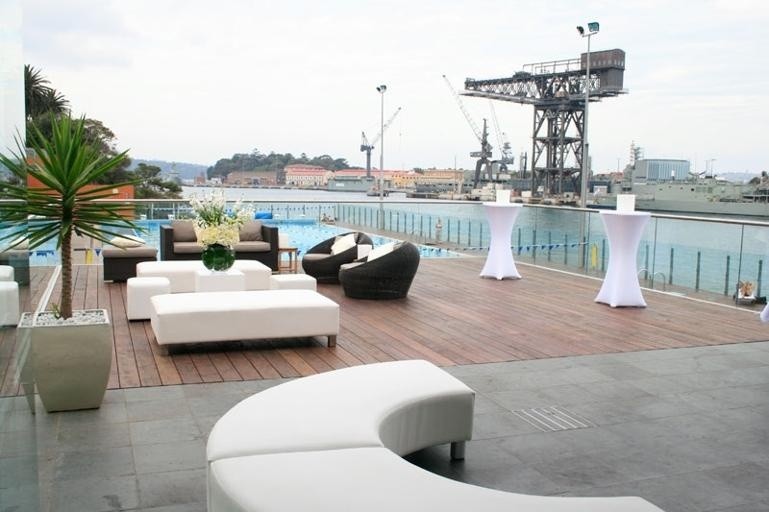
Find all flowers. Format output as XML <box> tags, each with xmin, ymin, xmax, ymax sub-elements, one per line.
<box><xmin>188</xmin><ymin>181</ymin><xmax>255</xmax><ymax>251</ymax></box>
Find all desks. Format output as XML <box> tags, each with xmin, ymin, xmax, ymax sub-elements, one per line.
<box><xmin>192</xmin><ymin>267</ymin><xmax>246</xmax><ymax>292</ymax></box>
<box><xmin>479</xmin><ymin>201</ymin><xmax>524</xmax><ymax>283</ymax></box>
<box><xmin>595</xmin><ymin>209</ymin><xmax>653</xmax><ymax>309</ymax></box>
<box><xmin>277</xmin><ymin>246</ymin><xmax>298</xmax><ymax>273</ymax></box>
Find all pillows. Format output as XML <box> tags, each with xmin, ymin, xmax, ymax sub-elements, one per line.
<box><xmin>9</xmin><ymin>236</ymin><xmax>28</xmax><ymax>250</ymax></box>
<box><xmin>109</xmin><ymin>234</ymin><xmax>145</xmax><ymax>248</ymax></box>
<box><xmin>331</xmin><ymin>233</ymin><xmax>357</xmax><ymax>256</ymax></box>
<box><xmin>367</xmin><ymin>243</ymin><xmax>394</xmax><ymax>265</ymax></box>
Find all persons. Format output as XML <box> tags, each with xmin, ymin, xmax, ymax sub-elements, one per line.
<box><xmin>759</xmin><ymin>302</ymin><xmax>769</xmax><ymax>321</ymax></box>
<box><xmin>733</xmin><ymin>279</ymin><xmax>758</xmax><ymax>300</ymax></box>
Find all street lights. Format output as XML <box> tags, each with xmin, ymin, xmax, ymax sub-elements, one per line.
<box><xmin>376</xmin><ymin>85</ymin><xmax>386</xmax><ymax>229</ymax></box>
<box><xmin>577</xmin><ymin>21</ymin><xmax>600</xmax><ymax>268</ymax></box>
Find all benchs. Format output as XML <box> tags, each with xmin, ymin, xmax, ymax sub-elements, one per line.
<box><xmin>150</xmin><ymin>290</ymin><xmax>341</xmax><ymax>354</ymax></box>
<box><xmin>201</xmin><ymin>356</ymin><xmax>661</xmax><ymax>509</ymax></box>
<box><xmin>0</xmin><ymin>246</ymin><xmax>29</xmax><ymax>286</ymax></box>
<box><xmin>136</xmin><ymin>260</ymin><xmax>273</xmax><ymax>292</ymax></box>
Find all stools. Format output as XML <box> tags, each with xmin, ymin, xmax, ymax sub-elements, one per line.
<box><xmin>100</xmin><ymin>245</ymin><xmax>156</xmax><ymax>284</ymax></box>
<box><xmin>274</xmin><ymin>272</ymin><xmax>319</xmax><ymax>289</ymax></box>
<box><xmin>124</xmin><ymin>277</ymin><xmax>169</xmax><ymax>323</ymax></box>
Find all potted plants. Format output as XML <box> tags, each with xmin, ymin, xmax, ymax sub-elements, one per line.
<box><xmin>1</xmin><ymin>97</ymin><xmax>155</xmax><ymax>414</ymax></box>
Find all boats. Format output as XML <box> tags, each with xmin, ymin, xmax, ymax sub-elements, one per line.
<box><xmin>366</xmin><ymin>185</ymin><xmax>389</xmax><ymax>196</ymax></box>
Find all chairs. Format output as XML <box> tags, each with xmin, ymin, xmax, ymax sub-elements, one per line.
<box><xmin>0</xmin><ymin>264</ymin><xmax>21</xmax><ymax>328</ymax></box>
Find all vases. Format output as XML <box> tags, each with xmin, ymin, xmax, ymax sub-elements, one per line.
<box><xmin>199</xmin><ymin>240</ymin><xmax>235</xmax><ymax>270</ymax></box>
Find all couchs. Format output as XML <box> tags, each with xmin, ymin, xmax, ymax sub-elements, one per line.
<box><xmin>160</xmin><ymin>217</ymin><xmax>280</xmax><ymax>270</ymax></box>
<box><xmin>303</xmin><ymin>228</ymin><xmax>374</xmax><ymax>279</ymax></box>
<box><xmin>338</xmin><ymin>241</ymin><xmax>422</xmax><ymax>301</ymax></box>
